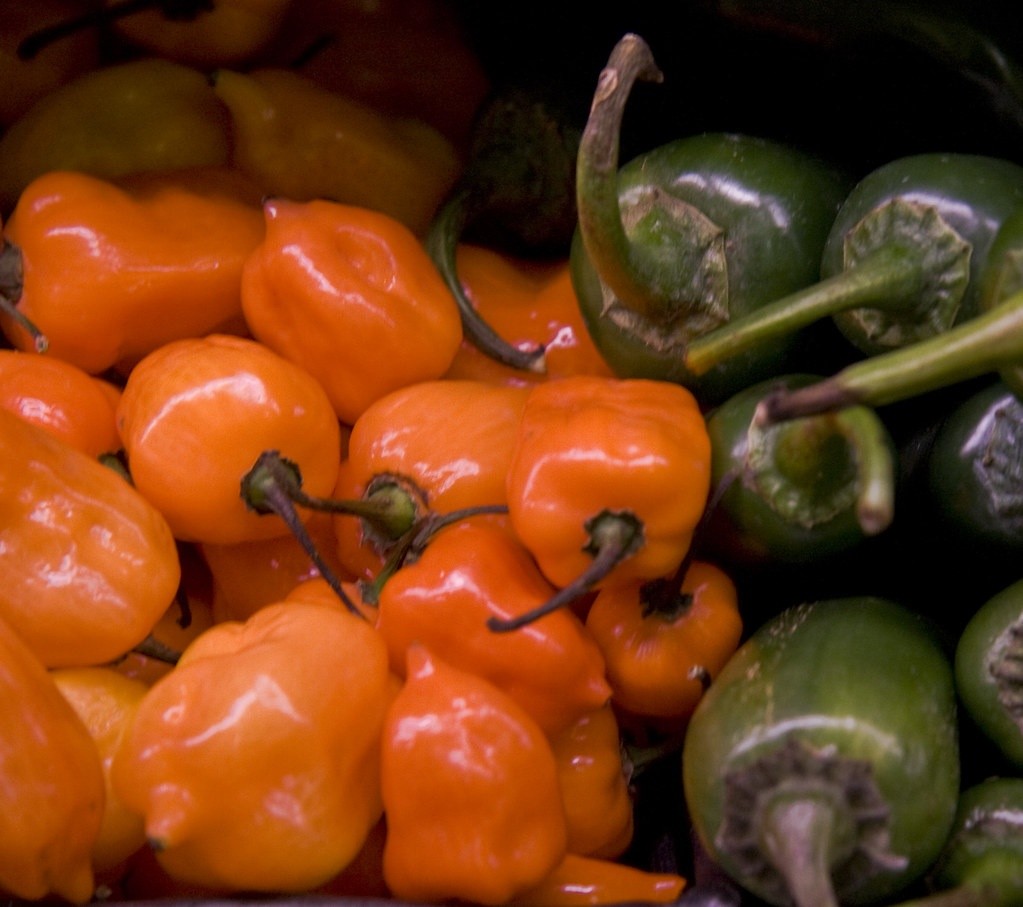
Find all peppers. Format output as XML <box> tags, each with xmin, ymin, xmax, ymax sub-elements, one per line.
<box><xmin>0</xmin><ymin>0</ymin><xmax>1023</xmax><ymax>907</ymax></box>
